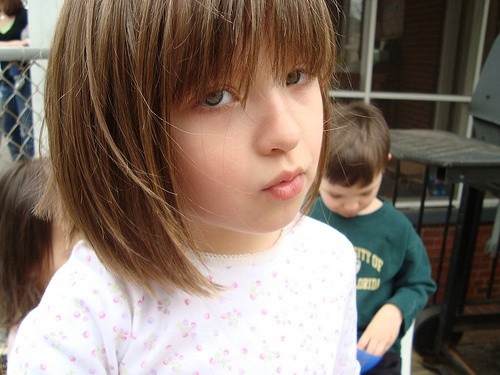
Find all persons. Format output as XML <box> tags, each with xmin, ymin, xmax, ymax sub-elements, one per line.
<box><xmin>12</xmin><ymin>0</ymin><xmax>362</xmax><ymax>373</ymax></box>
<box><xmin>300</xmin><ymin>100</ymin><xmax>437</xmax><ymax>375</ymax></box>
<box><xmin>1</xmin><ymin>0</ymin><xmax>38</xmax><ymax>160</ymax></box>
<box><xmin>0</xmin><ymin>155</ymin><xmax>91</xmax><ymax>374</ymax></box>
